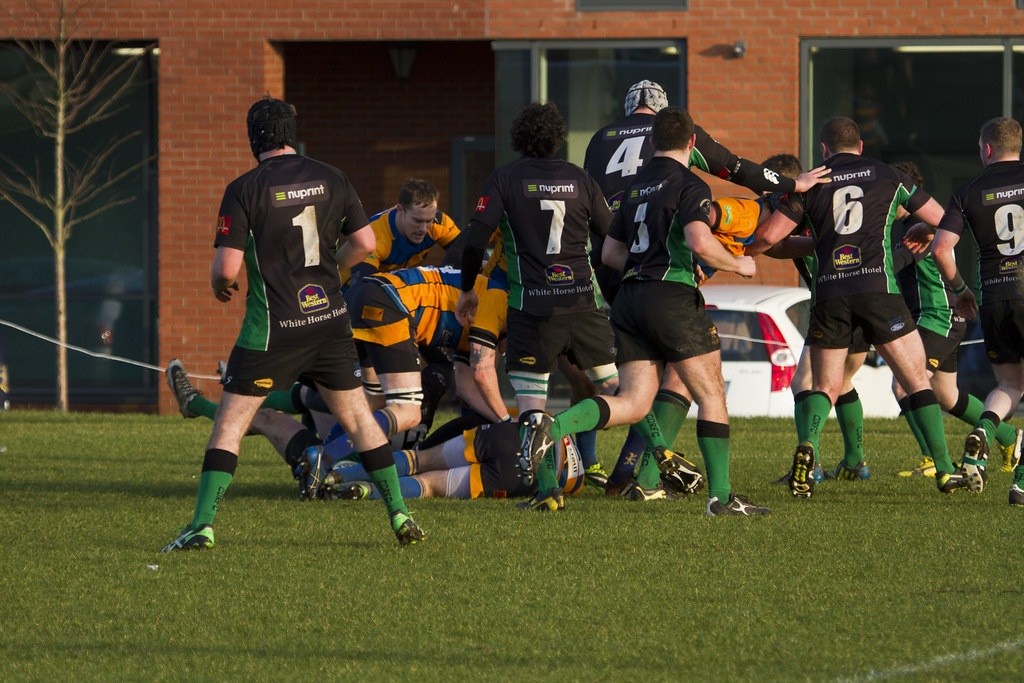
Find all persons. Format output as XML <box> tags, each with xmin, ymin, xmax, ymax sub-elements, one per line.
<box><xmin>158</xmin><ymin>98</ymin><xmax>426</xmax><ymax>548</ymax></box>
<box><xmin>168</xmin><ymin>117</ymin><xmax>1024</xmax><ymax>512</ymax></box>
<box><xmin>583</xmin><ymin>78</ymin><xmax>832</xmax><ymax>502</ymax></box>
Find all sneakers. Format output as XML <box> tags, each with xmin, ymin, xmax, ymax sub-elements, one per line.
<box><xmin>516</xmin><ymin>412</ymin><xmax>556</xmax><ymax>486</ymax></box>
<box><xmin>707</xmin><ymin>494</ymin><xmax>772</xmax><ymax>517</ymax></box>
<box><xmin>998</xmin><ymin>427</ymin><xmax>1024</xmax><ymax>473</ymax></box>
<box><xmin>514</xmin><ymin>486</ymin><xmax>566</xmax><ymax>512</ymax></box>
<box><xmin>322</xmin><ymin>471</ymin><xmax>338</xmax><ymax>487</ymax></box>
<box><xmin>301</xmin><ymin>445</ymin><xmax>329</xmax><ymax>500</ymax></box>
<box><xmin>771</xmin><ymin>464</ymin><xmax>826</xmax><ymax>485</ymax></box>
<box><xmin>604</xmin><ymin>475</ymin><xmax>637</xmax><ymax>497</ymax></box>
<box><xmin>788</xmin><ymin>441</ymin><xmax>815</xmax><ymax>497</ymax></box>
<box><xmin>215</xmin><ymin>360</ymin><xmax>228</xmax><ymax>385</ymax></box>
<box><xmin>325</xmin><ymin>481</ymin><xmax>370</xmax><ymax>501</ymax></box>
<box><xmin>624</xmin><ymin>482</ymin><xmax>667</xmax><ymax>502</ymax></box>
<box><xmin>390</xmin><ymin>511</ymin><xmax>426</xmax><ymax>546</ymax></box>
<box><xmin>1008</xmin><ymin>483</ymin><xmax>1024</xmax><ymax>506</ymax></box>
<box><xmin>959</xmin><ymin>428</ymin><xmax>988</xmax><ymax>493</ymax></box>
<box><xmin>897</xmin><ymin>456</ymin><xmax>937</xmax><ymax>478</ymax></box>
<box><xmin>823</xmin><ymin>459</ymin><xmax>871</xmax><ymax>481</ymax></box>
<box><xmin>935</xmin><ymin>462</ymin><xmax>989</xmax><ymax>494</ymax></box>
<box><xmin>160</xmin><ymin>524</ymin><xmax>214</xmax><ymax>553</ymax></box>
<box><xmin>585</xmin><ymin>462</ymin><xmax>611</xmax><ymax>491</ymax></box>
<box><xmin>976</xmin><ymin>440</ymin><xmax>990</xmax><ymax>485</ymax></box>
<box><xmin>165</xmin><ymin>357</ymin><xmax>204</xmax><ymax>419</ymax></box>
<box><xmin>651</xmin><ymin>445</ymin><xmax>706</xmax><ymax>493</ymax></box>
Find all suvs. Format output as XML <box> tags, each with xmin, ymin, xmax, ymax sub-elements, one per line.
<box><xmin>684</xmin><ymin>283</ymin><xmax>905</xmax><ymax>418</ymax></box>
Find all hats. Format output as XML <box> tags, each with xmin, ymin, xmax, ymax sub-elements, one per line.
<box><xmin>624</xmin><ymin>79</ymin><xmax>668</xmax><ymax>118</ymax></box>
